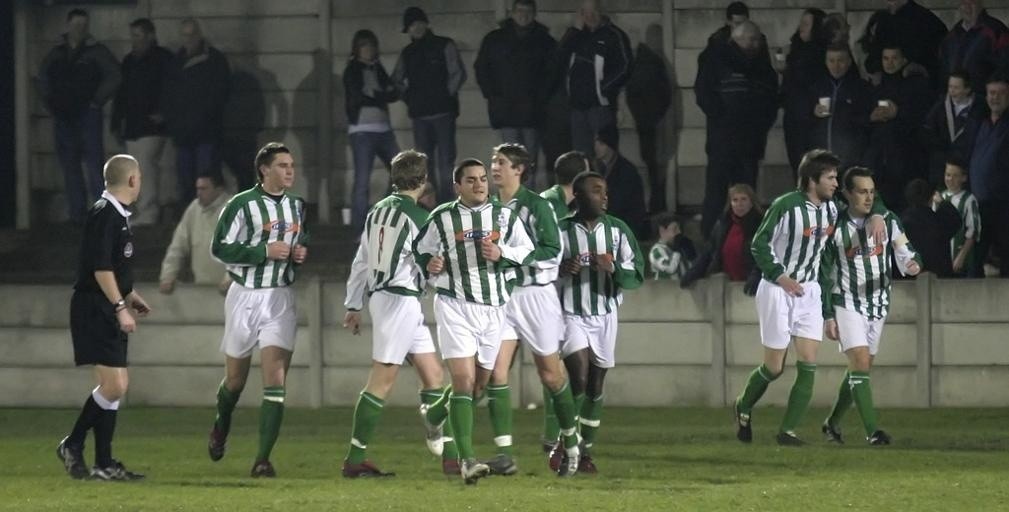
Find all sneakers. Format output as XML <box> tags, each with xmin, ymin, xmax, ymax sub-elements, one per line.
<box><xmin>208</xmin><ymin>425</ymin><xmax>229</xmax><ymax>461</ymax></box>
<box><xmin>56</xmin><ymin>435</ymin><xmax>91</xmax><ymax>480</ymax></box>
<box><xmin>543</xmin><ymin>439</ymin><xmax>598</xmax><ymax>477</ymax></box>
<box><xmin>342</xmin><ymin>462</ymin><xmax>396</xmax><ymax>478</ymax></box>
<box><xmin>776</xmin><ymin>431</ymin><xmax>804</xmax><ymax>446</ymax></box>
<box><xmin>823</xmin><ymin>419</ymin><xmax>844</xmax><ymax>445</ymax></box>
<box><xmin>420</xmin><ymin>403</ymin><xmax>518</xmax><ymax>486</ymax></box>
<box><xmin>733</xmin><ymin>397</ymin><xmax>752</xmax><ymax>443</ymax></box>
<box><xmin>89</xmin><ymin>458</ymin><xmax>145</xmax><ymax>480</ymax></box>
<box><xmin>251</xmin><ymin>460</ymin><xmax>274</xmax><ymax>478</ymax></box>
<box><xmin>866</xmin><ymin>430</ymin><xmax>890</xmax><ymax>446</ymax></box>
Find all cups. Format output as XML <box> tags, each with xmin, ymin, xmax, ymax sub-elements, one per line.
<box><xmin>341</xmin><ymin>207</ymin><xmax>352</xmax><ymax>225</ymax></box>
<box><xmin>878</xmin><ymin>99</ymin><xmax>889</xmax><ymax>107</ymax></box>
<box><xmin>819</xmin><ymin>98</ymin><xmax>832</xmax><ymax>115</ymax></box>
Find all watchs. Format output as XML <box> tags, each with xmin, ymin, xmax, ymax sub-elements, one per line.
<box><xmin>111</xmin><ymin>298</ymin><xmax>127</xmax><ymax>309</ymax></box>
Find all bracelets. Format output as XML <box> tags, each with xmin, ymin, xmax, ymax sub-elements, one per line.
<box><xmin>115</xmin><ymin>306</ymin><xmax>129</xmax><ymax>314</ymax></box>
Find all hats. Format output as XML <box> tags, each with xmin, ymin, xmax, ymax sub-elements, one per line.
<box><xmin>401</xmin><ymin>6</ymin><xmax>429</xmax><ymax>32</ymax></box>
<box><xmin>594</xmin><ymin>117</ymin><xmax>618</xmax><ymax>146</ymax></box>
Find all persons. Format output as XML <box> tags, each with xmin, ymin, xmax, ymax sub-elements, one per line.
<box><xmin>818</xmin><ymin>164</ymin><xmax>925</xmax><ymax>449</ymax></box>
<box><xmin>338</xmin><ymin>147</ymin><xmax>460</xmax><ymax>483</ymax></box>
<box><xmin>55</xmin><ymin>153</ymin><xmax>156</xmax><ymax>484</ymax></box>
<box><xmin>539</xmin><ymin>148</ymin><xmax>591</xmax><ymax>458</ymax></box>
<box><xmin>729</xmin><ymin>147</ymin><xmax>889</xmax><ymax>449</ymax></box>
<box><xmin>29</xmin><ymin>2</ymin><xmax>1008</xmax><ymax>299</ymax></box>
<box><xmin>410</xmin><ymin>155</ymin><xmax>539</xmax><ymax>489</ymax></box>
<box><xmin>548</xmin><ymin>170</ymin><xmax>648</xmax><ymax>479</ymax></box>
<box><xmin>481</xmin><ymin>141</ymin><xmax>587</xmax><ymax>480</ymax></box>
<box><xmin>205</xmin><ymin>138</ymin><xmax>316</xmax><ymax>480</ymax></box>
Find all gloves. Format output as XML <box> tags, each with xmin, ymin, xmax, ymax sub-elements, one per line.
<box><xmin>680</xmin><ymin>265</ymin><xmax>697</xmax><ymax>287</ymax></box>
<box><xmin>744</xmin><ymin>268</ymin><xmax>759</xmax><ymax>295</ymax></box>
